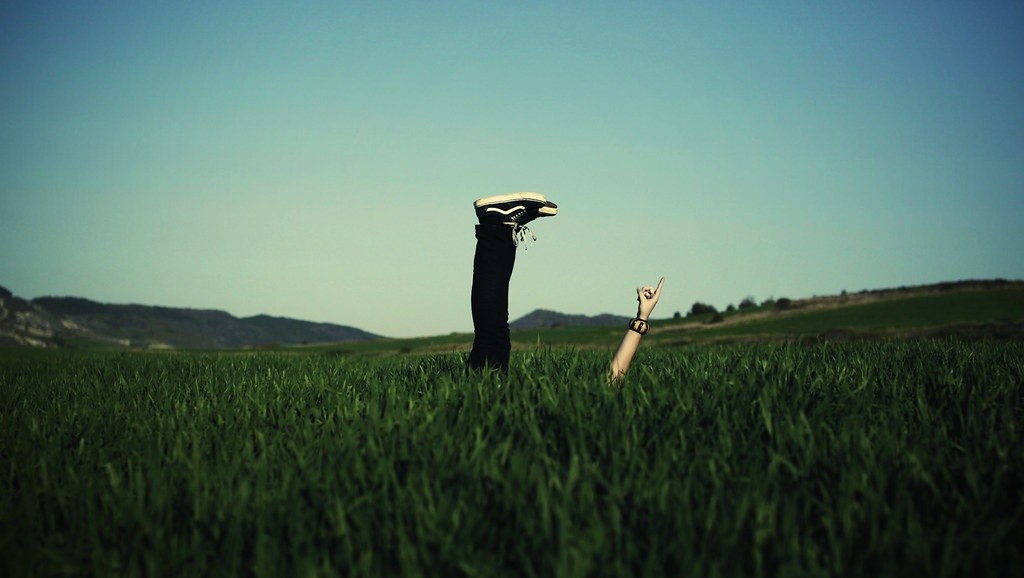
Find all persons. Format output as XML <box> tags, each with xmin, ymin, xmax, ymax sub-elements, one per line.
<box><xmin>467</xmin><ymin>192</ymin><xmax>665</xmax><ymax>382</ymax></box>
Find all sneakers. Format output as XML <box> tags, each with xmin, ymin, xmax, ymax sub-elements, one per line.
<box><xmin>473</xmin><ymin>192</ymin><xmax>558</xmax><ymax>250</ymax></box>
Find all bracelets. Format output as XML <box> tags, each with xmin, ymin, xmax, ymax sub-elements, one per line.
<box><xmin>629</xmin><ymin>318</ymin><xmax>649</xmax><ymax>335</ymax></box>
